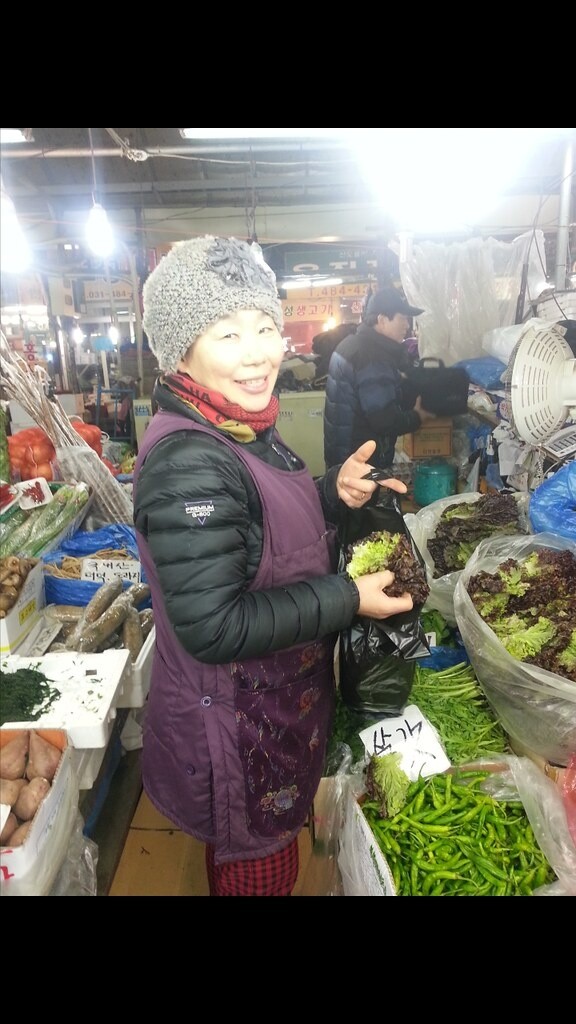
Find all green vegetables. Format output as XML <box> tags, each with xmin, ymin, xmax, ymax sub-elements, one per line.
<box><xmin>0</xmin><ymin>661</ymin><xmax>62</xmax><ymax>726</ymax></box>
<box><xmin>325</xmin><ymin>659</ymin><xmax>513</xmax><ymax>816</ymax></box>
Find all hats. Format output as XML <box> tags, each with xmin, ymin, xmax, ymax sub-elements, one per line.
<box><xmin>366</xmin><ymin>288</ymin><xmax>425</xmax><ymax>316</ymax></box>
<box><xmin>141</xmin><ymin>234</ymin><xmax>285</xmax><ymax>377</ymax></box>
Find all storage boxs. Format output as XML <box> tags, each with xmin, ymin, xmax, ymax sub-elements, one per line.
<box><xmin>10</xmin><ymin>421</ymin><xmax>38</xmax><ymax>436</ymax></box>
<box><xmin>0</xmin><ymin>727</ymin><xmax>81</xmax><ymax>896</ymax></box>
<box><xmin>9</xmin><ymin>401</ymin><xmax>37</xmax><ymax>421</ymax></box>
<box><xmin>28</xmin><ymin>621</ymin><xmax>156</xmax><ymax>709</ymax></box>
<box><xmin>337</xmin><ymin>761</ymin><xmax>567</xmax><ymax>897</ymax></box>
<box><xmin>57</xmin><ymin>390</ymin><xmax>85</xmax><ymax>413</ymax></box>
<box><xmin>0</xmin><ymin>557</ymin><xmax>47</xmax><ymax>656</ymax></box>
<box><xmin>311</xmin><ymin>776</ymin><xmax>361</xmax><ymax>857</ymax></box>
<box><xmin>402</xmin><ymin>416</ymin><xmax>453</xmax><ymax>460</ymax></box>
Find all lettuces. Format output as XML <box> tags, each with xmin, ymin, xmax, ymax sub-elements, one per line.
<box><xmin>346</xmin><ymin>487</ymin><xmax>576</xmax><ymax>685</ymax></box>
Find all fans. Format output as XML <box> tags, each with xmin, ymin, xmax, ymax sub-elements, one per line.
<box><xmin>505</xmin><ymin>325</ymin><xmax>576</xmax><ymax>447</ymax></box>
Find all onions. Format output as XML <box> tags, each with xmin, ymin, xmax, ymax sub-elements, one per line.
<box><xmin>5</xmin><ymin>421</ymin><xmax>102</xmax><ymax>480</ymax></box>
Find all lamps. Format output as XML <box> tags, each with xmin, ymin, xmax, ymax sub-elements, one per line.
<box><xmin>323</xmin><ymin>298</ymin><xmax>337</xmax><ymax>331</ymax></box>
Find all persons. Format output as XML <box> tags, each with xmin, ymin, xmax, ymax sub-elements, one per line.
<box><xmin>324</xmin><ymin>289</ymin><xmax>436</xmax><ymax>472</ymax></box>
<box><xmin>132</xmin><ymin>235</ymin><xmax>412</xmax><ymax>897</ymax></box>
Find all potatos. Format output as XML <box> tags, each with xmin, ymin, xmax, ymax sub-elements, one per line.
<box><xmin>0</xmin><ymin>728</ymin><xmax>61</xmax><ymax>847</ymax></box>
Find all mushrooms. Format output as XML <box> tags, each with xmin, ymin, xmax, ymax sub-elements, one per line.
<box><xmin>0</xmin><ymin>555</ymin><xmax>32</xmax><ymax>621</ymax></box>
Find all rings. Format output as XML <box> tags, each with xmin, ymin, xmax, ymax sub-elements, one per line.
<box><xmin>360</xmin><ymin>492</ymin><xmax>366</xmax><ymax>500</ymax></box>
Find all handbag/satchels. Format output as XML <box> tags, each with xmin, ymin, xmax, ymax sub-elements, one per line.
<box><xmin>337</xmin><ymin>467</ymin><xmax>429</xmax><ymax>719</ymax></box>
<box><xmin>402</xmin><ymin>357</ymin><xmax>471</xmax><ymax>417</ymax></box>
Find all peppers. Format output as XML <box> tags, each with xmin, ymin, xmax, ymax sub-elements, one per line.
<box><xmin>358</xmin><ymin>760</ymin><xmax>555</xmax><ymax>896</ymax></box>
<box><xmin>0</xmin><ymin>481</ymin><xmax>46</xmax><ymax>508</ymax></box>
